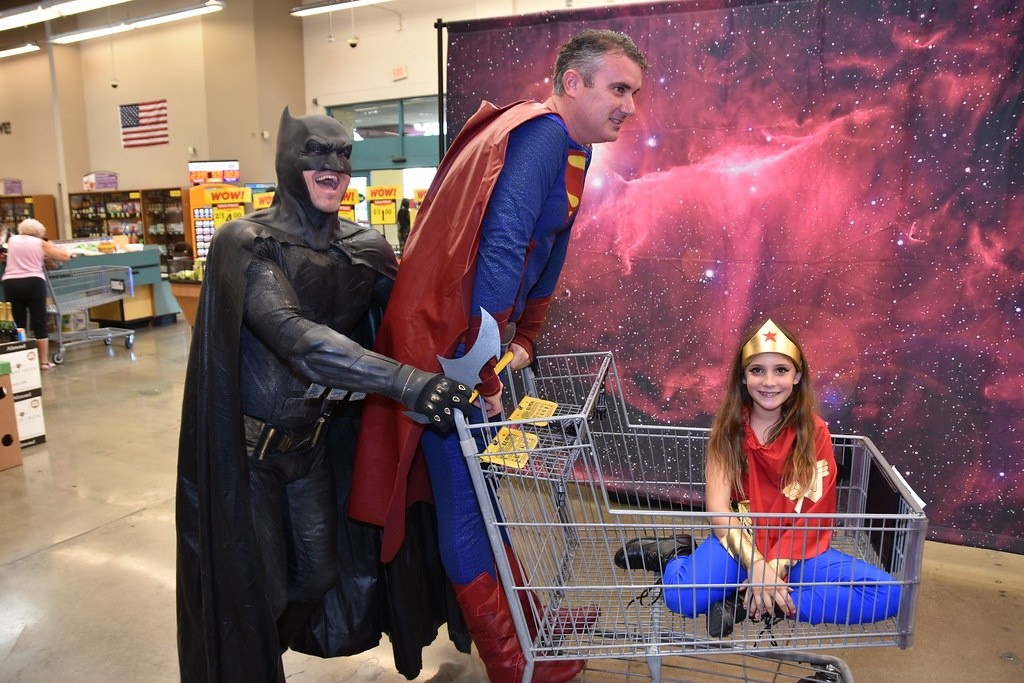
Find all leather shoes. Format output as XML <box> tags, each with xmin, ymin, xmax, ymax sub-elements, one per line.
<box><xmin>705</xmin><ymin>596</ymin><xmax>752</xmax><ymax>637</ymax></box>
<box><xmin>614</xmin><ymin>534</ymin><xmax>696</xmax><ymax>575</ymax></box>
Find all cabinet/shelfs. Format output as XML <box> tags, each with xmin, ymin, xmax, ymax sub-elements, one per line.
<box><xmin>66</xmin><ymin>189</ymin><xmax>193</xmax><ymax>280</ymax></box>
<box><xmin>0</xmin><ymin>194</ymin><xmax>59</xmax><ymax>247</ymax></box>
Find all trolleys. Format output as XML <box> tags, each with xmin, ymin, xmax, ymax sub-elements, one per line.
<box><xmin>449</xmin><ymin>335</ymin><xmax>930</xmax><ymax>683</ymax></box>
<box><xmin>42</xmin><ymin>263</ymin><xmax>137</xmax><ymax>365</ymax></box>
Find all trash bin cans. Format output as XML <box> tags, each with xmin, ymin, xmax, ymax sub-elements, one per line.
<box><xmin>172</xmin><ymin>241</ymin><xmax>194</xmax><ymax>272</ymax></box>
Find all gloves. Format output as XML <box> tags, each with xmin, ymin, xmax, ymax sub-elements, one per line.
<box><xmin>416</xmin><ymin>374</ymin><xmax>473</xmax><ymax>435</ymax></box>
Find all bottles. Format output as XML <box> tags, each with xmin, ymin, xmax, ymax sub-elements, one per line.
<box><xmin>72</xmin><ymin>199</ymin><xmax>184</xmax><ymax>238</ymax></box>
<box><xmin>0</xmin><ymin>302</ymin><xmax>18</xmax><ymax>355</ymax></box>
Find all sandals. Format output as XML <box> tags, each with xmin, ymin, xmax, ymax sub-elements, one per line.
<box><xmin>41</xmin><ymin>362</ymin><xmax>55</xmax><ymax>371</ymax></box>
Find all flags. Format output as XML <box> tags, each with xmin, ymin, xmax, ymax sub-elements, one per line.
<box><xmin>119</xmin><ymin>99</ymin><xmax>170</xmax><ymax>148</ymax></box>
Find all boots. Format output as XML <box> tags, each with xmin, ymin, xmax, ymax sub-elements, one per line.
<box><xmin>493</xmin><ymin>543</ymin><xmax>603</xmax><ymax>634</ymax></box>
<box><xmin>453</xmin><ymin>564</ymin><xmax>585</xmax><ymax>683</ymax></box>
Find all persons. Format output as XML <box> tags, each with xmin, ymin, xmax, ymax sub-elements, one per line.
<box><xmin>346</xmin><ymin>28</ymin><xmax>645</xmax><ymax>683</ymax></box>
<box><xmin>175</xmin><ymin>102</ymin><xmax>470</xmax><ymax>683</ymax></box>
<box><xmin>2</xmin><ymin>216</ymin><xmax>71</xmax><ymax>371</ymax></box>
<box><xmin>613</xmin><ymin>318</ymin><xmax>903</xmax><ymax>636</ymax></box>
